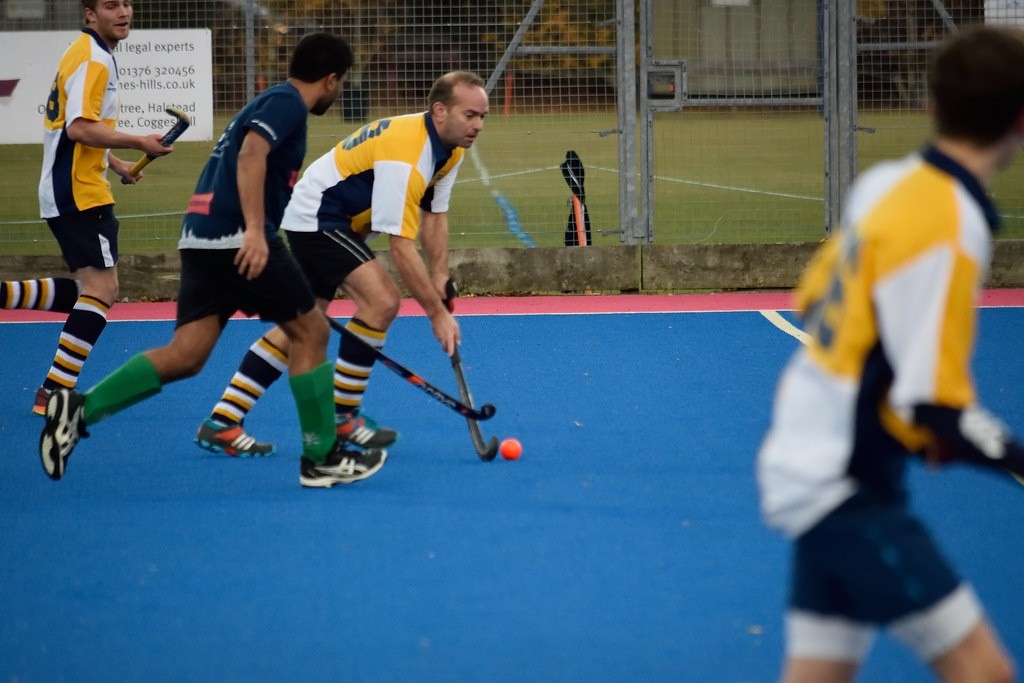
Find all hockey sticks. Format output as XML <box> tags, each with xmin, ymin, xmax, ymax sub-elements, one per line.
<box><xmin>326</xmin><ymin>313</ymin><xmax>500</xmax><ymax>422</ymax></box>
<box><xmin>441</xmin><ymin>274</ymin><xmax>499</xmax><ymax>462</ymax></box>
<box><xmin>120</xmin><ymin>107</ymin><xmax>191</xmax><ymax>186</ymax></box>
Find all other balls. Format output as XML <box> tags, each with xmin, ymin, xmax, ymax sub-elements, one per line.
<box><xmin>500</xmin><ymin>437</ymin><xmax>523</xmax><ymax>461</ymax></box>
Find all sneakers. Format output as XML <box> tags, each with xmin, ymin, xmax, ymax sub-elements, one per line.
<box><xmin>336</xmin><ymin>407</ymin><xmax>399</xmax><ymax>448</ymax></box>
<box><xmin>39</xmin><ymin>387</ymin><xmax>89</xmax><ymax>479</ymax></box>
<box><xmin>193</xmin><ymin>418</ymin><xmax>279</xmax><ymax>458</ymax></box>
<box><xmin>32</xmin><ymin>387</ymin><xmax>50</xmax><ymax>416</ymax></box>
<box><xmin>298</xmin><ymin>438</ymin><xmax>389</xmax><ymax>488</ymax></box>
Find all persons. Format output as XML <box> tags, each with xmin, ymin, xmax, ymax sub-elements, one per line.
<box><xmin>754</xmin><ymin>26</ymin><xmax>1024</xmax><ymax>683</ymax></box>
<box><xmin>39</xmin><ymin>32</ymin><xmax>390</xmax><ymax>489</ymax></box>
<box><xmin>194</xmin><ymin>71</ymin><xmax>487</xmax><ymax>458</ymax></box>
<box><xmin>1</xmin><ymin>0</ymin><xmax>175</xmax><ymax>417</ymax></box>
